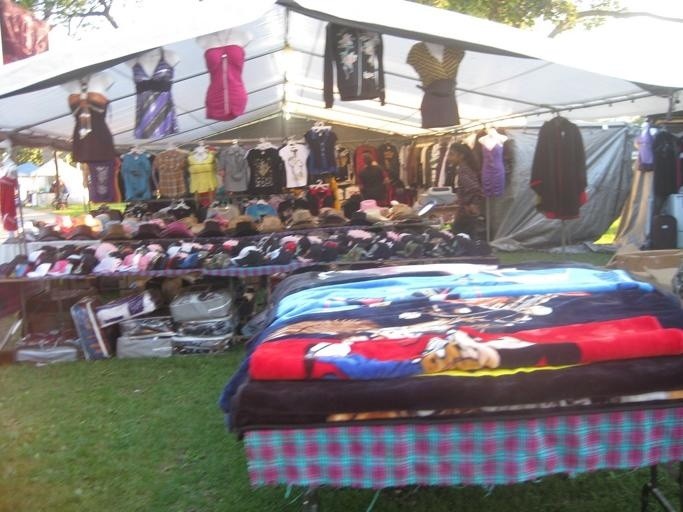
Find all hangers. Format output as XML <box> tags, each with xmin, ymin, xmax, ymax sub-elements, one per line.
<box><xmin>287</xmin><ymin>137</ymin><xmax>296</xmax><ymax>146</ymax></box>
<box><xmin>120</xmin><ymin>146</ymin><xmax>151</xmax><ymax>159</ymax></box>
<box><xmin>311</xmin><ymin>122</ymin><xmax>332</xmax><ymax>133</ymax></box>
<box><xmin>160</xmin><ymin>141</ymin><xmax>188</xmax><ymax>153</ymax></box>
<box><xmin>231</xmin><ymin>140</ymin><xmax>242</xmax><ymax>151</ymax></box>
<box><xmin>257</xmin><ymin>196</ymin><xmax>267</xmax><ymax>205</ymax></box>
<box><xmin>173</xmin><ymin>198</ymin><xmax>190</xmax><ymax>210</ymax></box>
<box><xmin>658</xmin><ymin>125</ymin><xmax>669</xmax><ymax>134</ymax></box>
<box><xmin>550</xmin><ymin>110</ymin><xmax>571</xmax><ymax>123</ymax></box>
<box><xmin>421</xmin><ymin>184</ymin><xmax>431</xmax><ymax>189</ymax></box>
<box><xmin>336</xmin><ymin>145</ymin><xmax>341</xmax><ymax>151</ymax></box>
<box><xmin>220</xmin><ymin>200</ymin><xmax>226</xmax><ymax>206</ymax></box>
<box><xmin>168</xmin><ymin>198</ymin><xmax>177</xmax><ymax>209</ymax></box>
<box><xmin>411</xmin><ymin>182</ymin><xmax>420</xmax><ymax>187</ymax></box>
<box><xmin>315</xmin><ymin>179</ymin><xmax>327</xmax><ymax>190</ymax></box>
<box><xmin>372</xmin><ymin>161</ymin><xmax>378</xmax><ymax>167</ymax></box>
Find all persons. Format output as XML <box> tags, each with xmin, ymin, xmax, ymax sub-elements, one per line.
<box><xmin>360</xmin><ymin>152</ymin><xmax>390</xmax><ymax>204</ymax></box>
<box><xmin>447</xmin><ymin>143</ymin><xmax>487</xmax><ymax>236</ymax></box>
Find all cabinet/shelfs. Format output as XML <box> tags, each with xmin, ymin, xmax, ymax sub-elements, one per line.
<box><xmin>236</xmin><ymin>263</ymin><xmax>683</xmax><ymax>485</ymax></box>
<box><xmin>0</xmin><ymin>196</ymin><xmax>501</xmax><ymax>358</ymax></box>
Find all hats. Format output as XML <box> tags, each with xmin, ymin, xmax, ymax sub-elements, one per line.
<box><xmin>255</xmin><ymin>216</ymin><xmax>287</xmax><ymax>233</ymax></box>
<box><xmin>102</xmin><ymin>226</ymin><xmax>129</xmax><ymax>241</ymax></box>
<box><xmin>37</xmin><ymin>226</ymin><xmax>64</xmax><ymax>241</ymax></box>
<box><xmin>130</xmin><ymin>223</ymin><xmax>162</xmax><ymax>239</ymax></box>
<box><xmin>365</xmin><ymin>208</ymin><xmax>388</xmax><ymax>221</ymax></box>
<box><xmin>68</xmin><ymin>225</ymin><xmax>96</xmax><ymax>240</ymax></box>
<box><xmin>357</xmin><ymin>199</ymin><xmax>388</xmax><ymax>211</ymax></box>
<box><xmin>287</xmin><ymin>209</ymin><xmax>319</xmax><ymax>229</ymax></box>
<box><xmin>344</xmin><ymin>212</ymin><xmax>373</xmax><ymax>226</ymax></box>
<box><xmin>197</xmin><ymin>221</ymin><xmax>226</xmax><ymax>237</ymax></box>
<box><xmin>161</xmin><ymin>221</ymin><xmax>195</xmax><ymax>238</ymax></box>
<box><xmin>231</xmin><ymin>221</ymin><xmax>258</xmax><ymax>237</ymax></box>
<box><xmin>319</xmin><ymin>213</ymin><xmax>345</xmax><ymax>228</ymax></box>
